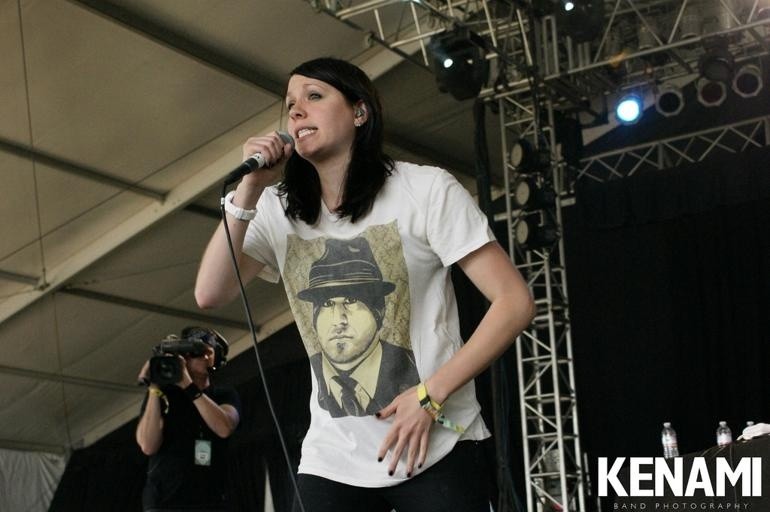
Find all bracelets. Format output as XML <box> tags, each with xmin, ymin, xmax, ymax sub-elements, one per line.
<box><xmin>148</xmin><ymin>386</ymin><xmax>164</xmax><ymax>398</ymax></box>
<box><xmin>184</xmin><ymin>382</ymin><xmax>202</xmax><ymax>400</ymax></box>
<box><xmin>416</xmin><ymin>379</ymin><xmax>448</xmax><ymax>418</ymax></box>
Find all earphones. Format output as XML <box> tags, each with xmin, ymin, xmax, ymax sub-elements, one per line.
<box><xmin>355</xmin><ymin>106</ymin><xmax>365</xmax><ymax>118</ymax></box>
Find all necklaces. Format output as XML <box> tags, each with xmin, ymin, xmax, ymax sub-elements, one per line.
<box><xmin>321</xmin><ymin>172</ymin><xmax>346</xmax><ymax>215</ymax></box>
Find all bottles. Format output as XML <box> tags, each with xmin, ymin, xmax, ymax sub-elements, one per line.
<box><xmin>661</xmin><ymin>422</ymin><xmax>679</xmax><ymax>458</ymax></box>
<box><xmin>716</xmin><ymin>421</ymin><xmax>732</xmax><ymax>446</ymax></box>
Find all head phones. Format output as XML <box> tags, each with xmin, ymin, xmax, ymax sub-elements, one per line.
<box><xmin>182</xmin><ymin>326</ymin><xmax>227</xmax><ymax>372</ymax></box>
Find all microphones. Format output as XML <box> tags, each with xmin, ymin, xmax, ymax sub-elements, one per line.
<box><xmin>207</xmin><ymin>366</ymin><xmax>221</xmax><ymax>377</ymax></box>
<box><xmin>224</xmin><ymin>131</ymin><xmax>295</xmax><ymax>186</ymax></box>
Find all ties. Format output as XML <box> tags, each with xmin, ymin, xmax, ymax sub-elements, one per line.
<box><xmin>338</xmin><ymin>374</ymin><xmax>361</xmax><ymax>419</ymax></box>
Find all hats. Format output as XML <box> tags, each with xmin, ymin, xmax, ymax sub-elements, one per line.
<box><xmin>297</xmin><ymin>236</ymin><xmax>397</xmax><ymax>303</ymax></box>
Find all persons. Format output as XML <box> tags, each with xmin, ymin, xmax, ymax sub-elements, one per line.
<box><xmin>135</xmin><ymin>327</ymin><xmax>241</xmax><ymax>512</ymax></box>
<box><xmin>297</xmin><ymin>238</ymin><xmax>421</xmax><ymax>418</ymax></box>
<box><xmin>194</xmin><ymin>58</ymin><xmax>536</xmax><ymax>512</ymax></box>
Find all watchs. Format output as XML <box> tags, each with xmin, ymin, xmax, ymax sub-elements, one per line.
<box><xmin>224</xmin><ymin>190</ymin><xmax>258</xmax><ymax>221</ymax></box>
